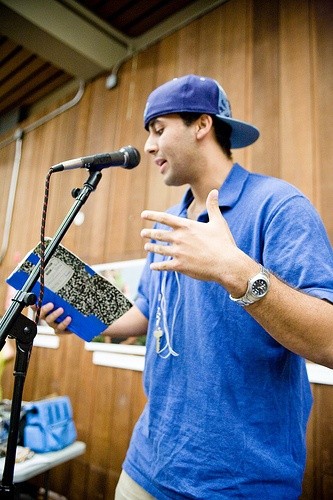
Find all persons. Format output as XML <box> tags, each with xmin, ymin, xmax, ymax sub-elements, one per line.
<box><xmin>32</xmin><ymin>73</ymin><xmax>333</xmax><ymax>500</ymax></box>
<box><xmin>104</xmin><ymin>281</ymin><xmax>138</xmax><ymax>345</ymax></box>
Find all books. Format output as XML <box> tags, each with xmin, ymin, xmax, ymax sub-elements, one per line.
<box><xmin>5</xmin><ymin>236</ymin><xmax>133</xmax><ymax>343</ymax></box>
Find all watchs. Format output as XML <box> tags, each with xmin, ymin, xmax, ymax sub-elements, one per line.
<box><xmin>229</xmin><ymin>263</ymin><xmax>270</xmax><ymax>307</ymax></box>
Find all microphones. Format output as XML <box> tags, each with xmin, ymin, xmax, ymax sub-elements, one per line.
<box><xmin>50</xmin><ymin>146</ymin><xmax>141</xmax><ymax>172</ymax></box>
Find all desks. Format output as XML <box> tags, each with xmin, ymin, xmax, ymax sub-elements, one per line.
<box><xmin>0</xmin><ymin>438</ymin><xmax>87</xmax><ymax>500</ymax></box>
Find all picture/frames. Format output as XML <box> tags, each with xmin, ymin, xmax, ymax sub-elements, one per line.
<box><xmin>85</xmin><ymin>259</ymin><xmax>150</xmax><ymax>357</ymax></box>
<box><xmin>27</xmin><ymin>306</ymin><xmax>55</xmax><ymax>335</ymax></box>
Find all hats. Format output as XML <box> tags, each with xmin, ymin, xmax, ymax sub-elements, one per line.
<box><xmin>142</xmin><ymin>74</ymin><xmax>259</xmax><ymax>150</ymax></box>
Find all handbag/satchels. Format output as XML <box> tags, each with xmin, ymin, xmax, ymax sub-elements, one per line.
<box><xmin>22</xmin><ymin>396</ymin><xmax>77</xmax><ymax>454</ymax></box>
